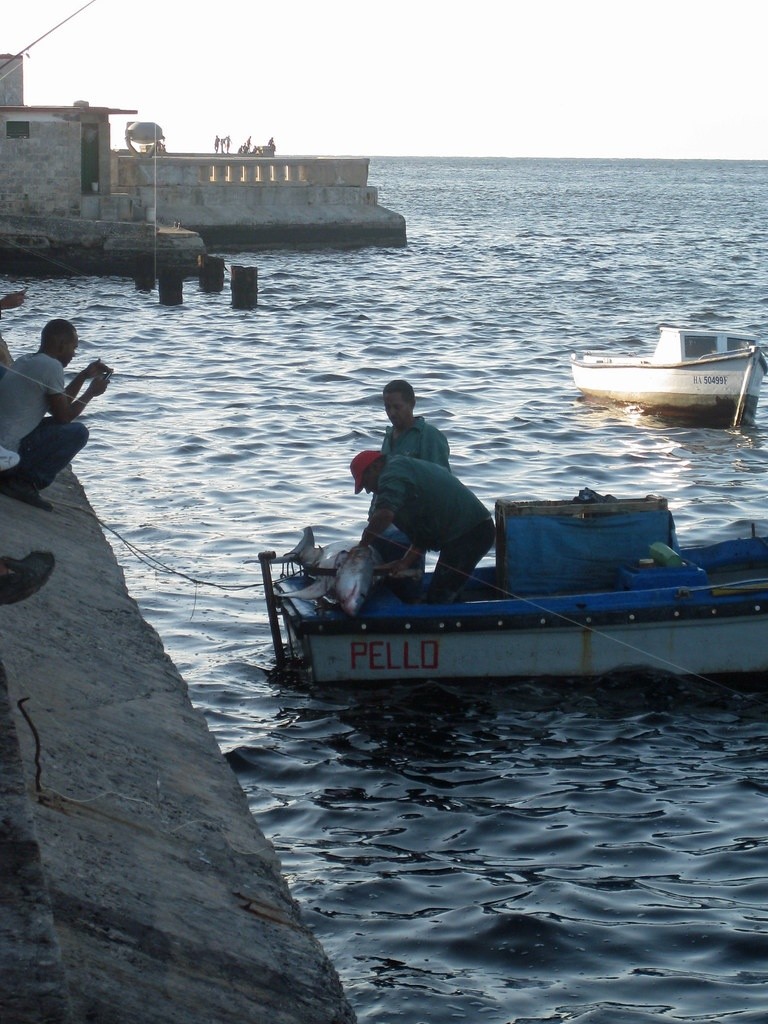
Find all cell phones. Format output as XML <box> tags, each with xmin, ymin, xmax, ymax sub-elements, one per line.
<box><xmin>104</xmin><ymin>372</ymin><xmax>112</xmax><ymax>380</ymax></box>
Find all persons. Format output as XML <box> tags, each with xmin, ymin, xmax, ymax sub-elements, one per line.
<box><xmin>367</xmin><ymin>380</ymin><xmax>451</xmax><ymax>605</ymax></box>
<box><xmin>350</xmin><ymin>450</ymin><xmax>496</xmax><ymax>605</ymax></box>
<box><xmin>0</xmin><ymin>292</ymin><xmax>29</xmax><ymax>367</ymax></box>
<box><xmin>0</xmin><ymin>550</ymin><xmax>55</xmax><ymax>607</ymax></box>
<box><xmin>0</xmin><ymin>319</ymin><xmax>114</xmax><ymax>512</ymax></box>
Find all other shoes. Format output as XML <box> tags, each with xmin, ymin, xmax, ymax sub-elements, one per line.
<box><xmin>0</xmin><ymin>551</ymin><xmax>55</xmax><ymax>605</ymax></box>
<box><xmin>0</xmin><ymin>475</ymin><xmax>54</xmax><ymax>512</ymax></box>
<box><xmin>0</xmin><ymin>446</ymin><xmax>20</xmax><ymax>472</ymax></box>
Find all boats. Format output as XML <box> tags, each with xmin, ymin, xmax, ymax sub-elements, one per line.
<box><xmin>568</xmin><ymin>326</ymin><xmax>768</xmax><ymax>430</ymax></box>
<box><xmin>259</xmin><ymin>486</ymin><xmax>768</xmax><ymax>685</ymax></box>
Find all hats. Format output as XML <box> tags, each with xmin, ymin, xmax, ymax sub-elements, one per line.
<box><xmin>350</xmin><ymin>450</ymin><xmax>383</xmax><ymax>494</ymax></box>
